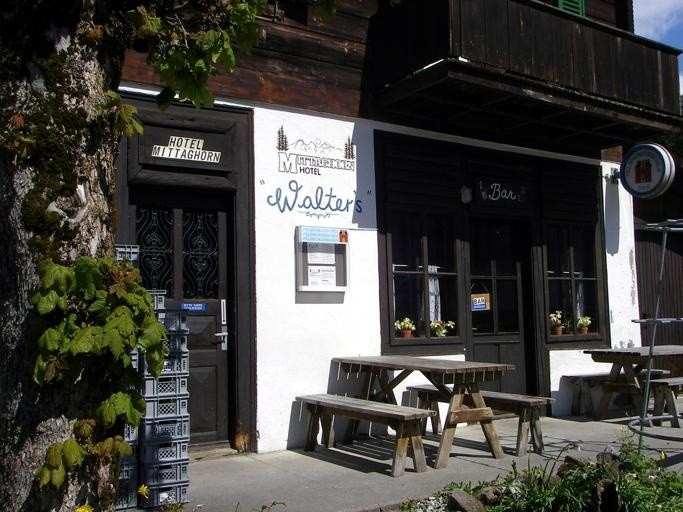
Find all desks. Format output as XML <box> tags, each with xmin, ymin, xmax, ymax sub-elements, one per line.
<box><xmin>584</xmin><ymin>345</ymin><xmax>683</xmax><ymax>427</ymax></box>
<box><xmin>336</xmin><ymin>356</ymin><xmax>516</xmax><ymax>468</ymax></box>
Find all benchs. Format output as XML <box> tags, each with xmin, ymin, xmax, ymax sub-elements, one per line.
<box><xmin>406</xmin><ymin>384</ymin><xmax>555</xmax><ymax>456</ymax></box>
<box><xmin>644</xmin><ymin>376</ymin><xmax>683</xmax><ymax>429</ymax></box>
<box><xmin>295</xmin><ymin>395</ymin><xmax>437</xmax><ymax>477</ymax></box>
<box><xmin>562</xmin><ymin>368</ymin><xmax>671</xmax><ymax>417</ymax></box>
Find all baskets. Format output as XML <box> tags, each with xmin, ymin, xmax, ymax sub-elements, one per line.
<box><xmin>113</xmin><ymin>243</ymin><xmax>189</xmax><ymax>509</ymax></box>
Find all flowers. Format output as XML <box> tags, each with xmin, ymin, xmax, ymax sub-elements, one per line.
<box><xmin>430</xmin><ymin>319</ymin><xmax>455</xmax><ymax>337</ymax></box>
<box><xmin>549</xmin><ymin>310</ymin><xmax>562</xmax><ymax>329</ymax></box>
<box><xmin>576</xmin><ymin>315</ymin><xmax>592</xmax><ymax>329</ymax></box>
<box><xmin>394</xmin><ymin>317</ymin><xmax>416</xmax><ymax>332</ymax></box>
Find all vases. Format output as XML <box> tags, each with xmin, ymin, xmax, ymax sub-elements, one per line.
<box><xmin>401</xmin><ymin>329</ymin><xmax>411</xmax><ymax>338</ymax></box>
<box><xmin>553</xmin><ymin>326</ymin><xmax>563</xmax><ymax>335</ymax></box>
<box><xmin>579</xmin><ymin>326</ymin><xmax>588</xmax><ymax>334</ymax></box>
